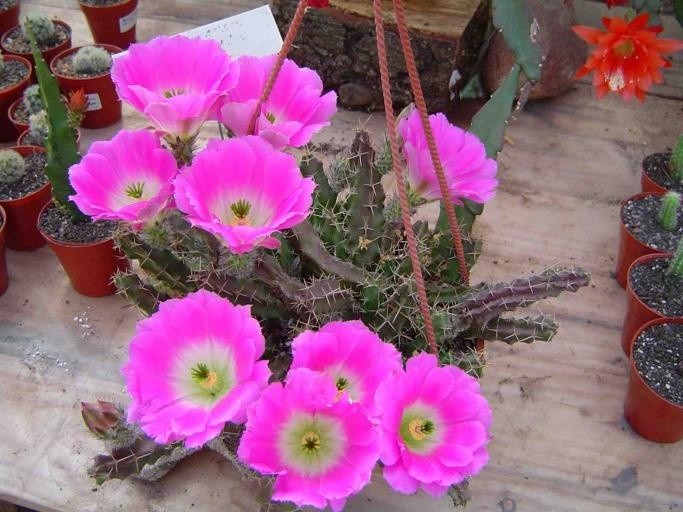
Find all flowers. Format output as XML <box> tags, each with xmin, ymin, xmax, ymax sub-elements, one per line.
<box><xmin>450</xmin><ymin>1</ymin><xmax>683</xmax><ymax>164</ymax></box>
<box><xmin>62</xmin><ymin>25</ymin><xmax>595</xmax><ymax>512</ymax></box>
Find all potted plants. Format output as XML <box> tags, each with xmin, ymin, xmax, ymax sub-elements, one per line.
<box><xmin>614</xmin><ymin>132</ymin><xmax>682</xmax><ymax>446</ymax></box>
<box><xmin>1</xmin><ymin>1</ymin><xmax>138</xmax><ymax>298</ymax></box>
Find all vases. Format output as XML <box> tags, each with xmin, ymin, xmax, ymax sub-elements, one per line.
<box><xmin>475</xmin><ymin>0</ymin><xmax>586</xmax><ymax>102</ymax></box>
